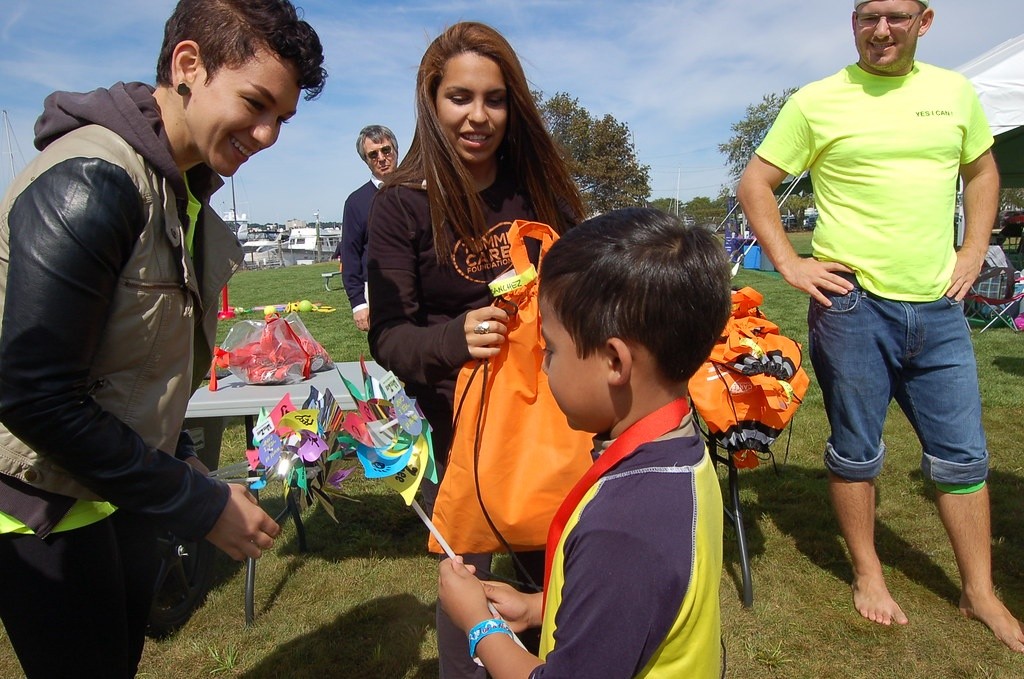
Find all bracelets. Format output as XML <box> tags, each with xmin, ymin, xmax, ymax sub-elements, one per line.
<box><xmin>468</xmin><ymin>620</ymin><xmax>513</xmax><ymax>667</ymax></box>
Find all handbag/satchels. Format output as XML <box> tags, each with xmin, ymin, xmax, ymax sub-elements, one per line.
<box><xmin>428</xmin><ymin>220</ymin><xmax>601</xmax><ymax>552</ymax></box>
<box><xmin>689</xmin><ymin>286</ymin><xmax>810</xmax><ymax>454</ymax></box>
<box><xmin>221</xmin><ymin>313</ymin><xmax>338</xmax><ymax>386</ymax></box>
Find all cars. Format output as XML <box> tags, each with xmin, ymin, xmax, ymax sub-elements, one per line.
<box><xmin>1000</xmin><ymin>211</ymin><xmax>1024</xmax><ymax>229</ymax></box>
<box><xmin>783</xmin><ymin>209</ymin><xmax>818</xmax><ymax>230</ymax></box>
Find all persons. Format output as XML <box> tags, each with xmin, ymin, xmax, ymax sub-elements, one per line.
<box><xmin>736</xmin><ymin>0</ymin><xmax>1024</xmax><ymax>654</ymax></box>
<box><xmin>0</xmin><ymin>0</ymin><xmax>327</xmax><ymax>679</ymax></box>
<box><xmin>366</xmin><ymin>22</ymin><xmax>591</xmax><ymax>678</ymax></box>
<box><xmin>341</xmin><ymin>125</ymin><xmax>400</xmax><ymax>331</ymax></box>
<box><xmin>438</xmin><ymin>208</ymin><xmax>732</xmax><ymax>679</ymax></box>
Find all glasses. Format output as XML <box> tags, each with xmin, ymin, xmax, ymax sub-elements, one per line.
<box><xmin>367</xmin><ymin>145</ymin><xmax>392</xmax><ymax>159</ymax></box>
<box><xmin>855</xmin><ymin>11</ymin><xmax>923</xmax><ymax>27</ymax></box>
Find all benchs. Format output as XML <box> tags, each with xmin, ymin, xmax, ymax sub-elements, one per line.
<box><xmin>321</xmin><ymin>269</ymin><xmax>341</xmax><ymax>292</ymax></box>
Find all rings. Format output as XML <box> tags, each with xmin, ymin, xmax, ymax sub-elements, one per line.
<box><xmin>474</xmin><ymin>322</ymin><xmax>490</xmax><ymax>334</ymax></box>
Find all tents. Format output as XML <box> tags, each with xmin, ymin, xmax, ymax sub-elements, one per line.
<box><xmin>949</xmin><ymin>34</ymin><xmax>1024</xmax><ymax>246</ymax></box>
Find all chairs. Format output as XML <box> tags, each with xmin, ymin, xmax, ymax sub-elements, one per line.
<box><xmin>963</xmin><ymin>244</ymin><xmax>1024</xmax><ymax>334</ymax></box>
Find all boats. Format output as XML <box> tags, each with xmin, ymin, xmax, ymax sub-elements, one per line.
<box><xmin>243</xmin><ymin>231</ymin><xmax>289</xmax><ymax>269</ymax></box>
<box><xmin>276</xmin><ymin>227</ymin><xmax>342</xmax><ymax>266</ymax></box>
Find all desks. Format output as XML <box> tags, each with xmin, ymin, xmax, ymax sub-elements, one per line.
<box><xmin>182</xmin><ymin>362</ymin><xmax>387</xmax><ymax>626</ymax></box>
